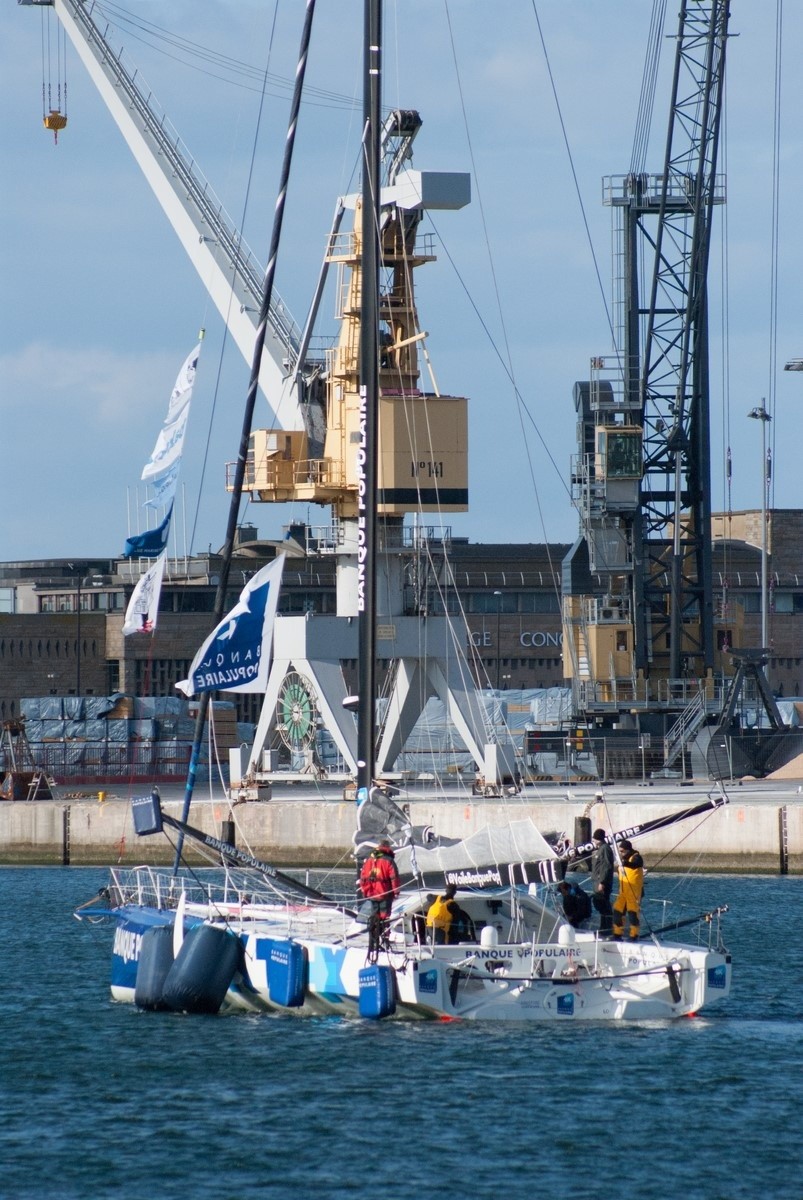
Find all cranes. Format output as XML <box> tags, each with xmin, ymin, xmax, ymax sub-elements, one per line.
<box><xmin>16</xmin><ymin>1</ymin><xmax>523</xmax><ymax>795</ymax></box>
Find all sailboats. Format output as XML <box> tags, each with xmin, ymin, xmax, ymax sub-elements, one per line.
<box><xmin>72</xmin><ymin>0</ymin><xmax>738</xmax><ymax>1033</ymax></box>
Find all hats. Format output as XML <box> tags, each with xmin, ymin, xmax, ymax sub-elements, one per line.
<box><xmin>593</xmin><ymin>828</ymin><xmax>605</xmax><ymax>843</ymax></box>
<box><xmin>620</xmin><ymin>840</ymin><xmax>632</xmax><ymax>850</ymax></box>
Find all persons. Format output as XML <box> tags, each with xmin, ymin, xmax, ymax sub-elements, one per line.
<box><xmin>358</xmin><ymin>838</ymin><xmax>400</xmax><ymax>950</ymax></box>
<box><xmin>589</xmin><ymin>828</ymin><xmax>614</xmax><ymax>936</ymax></box>
<box><xmin>556</xmin><ymin>881</ymin><xmax>592</xmax><ymax>928</ymax></box>
<box><xmin>607</xmin><ymin>840</ymin><xmax>643</xmax><ymax>941</ymax></box>
<box><xmin>425</xmin><ymin>882</ymin><xmax>461</xmax><ymax>945</ymax></box>
